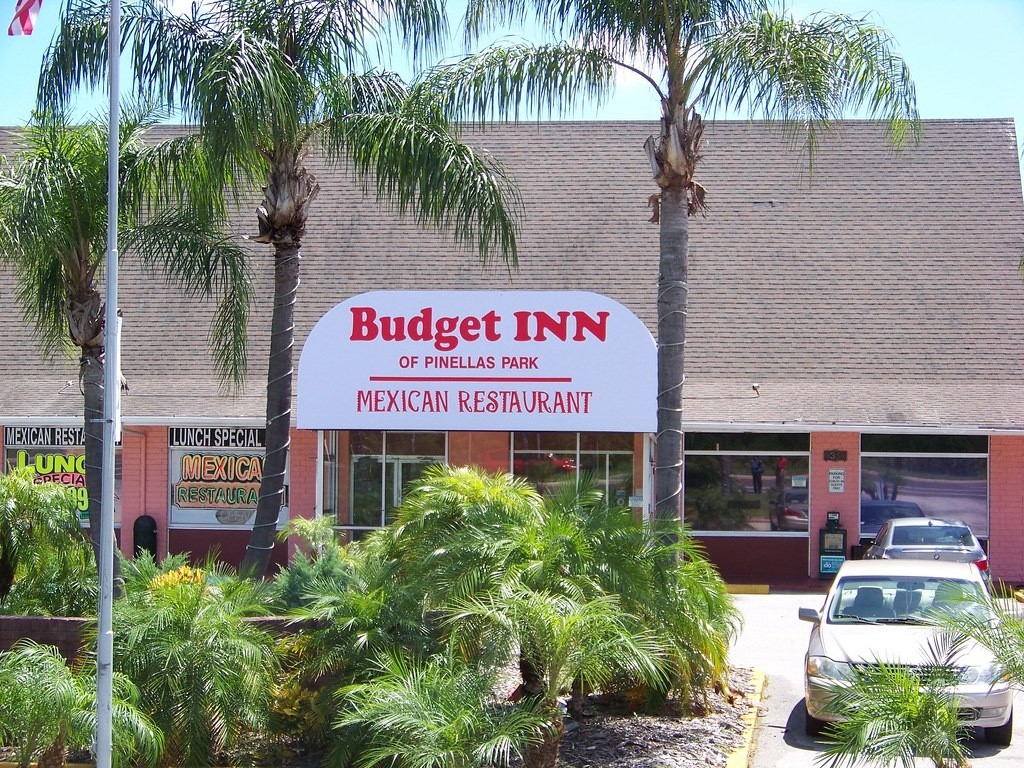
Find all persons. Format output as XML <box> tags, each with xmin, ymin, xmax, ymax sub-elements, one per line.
<box><xmin>775</xmin><ymin>457</ymin><xmax>788</xmax><ymax>489</ymax></box>
<box><xmin>751</xmin><ymin>456</ymin><xmax>765</xmax><ymax>493</ymax></box>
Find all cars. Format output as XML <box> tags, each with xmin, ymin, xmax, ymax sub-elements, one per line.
<box><xmin>798</xmin><ymin>560</ymin><xmax>1014</xmax><ymax>746</ymax></box>
<box><xmin>483</xmin><ymin>449</ymin><xmax>577</xmax><ymax>475</ymax></box>
<box><xmin>861</xmin><ymin>500</ymin><xmax>927</xmax><ymax>533</ymax></box>
<box><xmin>863</xmin><ymin>517</ymin><xmax>990</xmax><ymax>589</ymax></box>
<box><xmin>769</xmin><ymin>489</ymin><xmax>808</xmax><ymax>532</ymax></box>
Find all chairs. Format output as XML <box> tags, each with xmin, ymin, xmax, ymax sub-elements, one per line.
<box><xmin>841</xmin><ymin>588</ymin><xmax>896</xmax><ymax>618</ymax></box>
<box><xmin>921</xmin><ymin>582</ymin><xmax>972</xmax><ymax>619</ymax></box>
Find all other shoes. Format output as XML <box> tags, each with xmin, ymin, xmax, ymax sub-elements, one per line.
<box><xmin>754</xmin><ymin>489</ymin><xmax>757</xmax><ymax>493</ymax></box>
<box><xmin>758</xmin><ymin>489</ymin><xmax>761</xmax><ymax>493</ymax></box>
